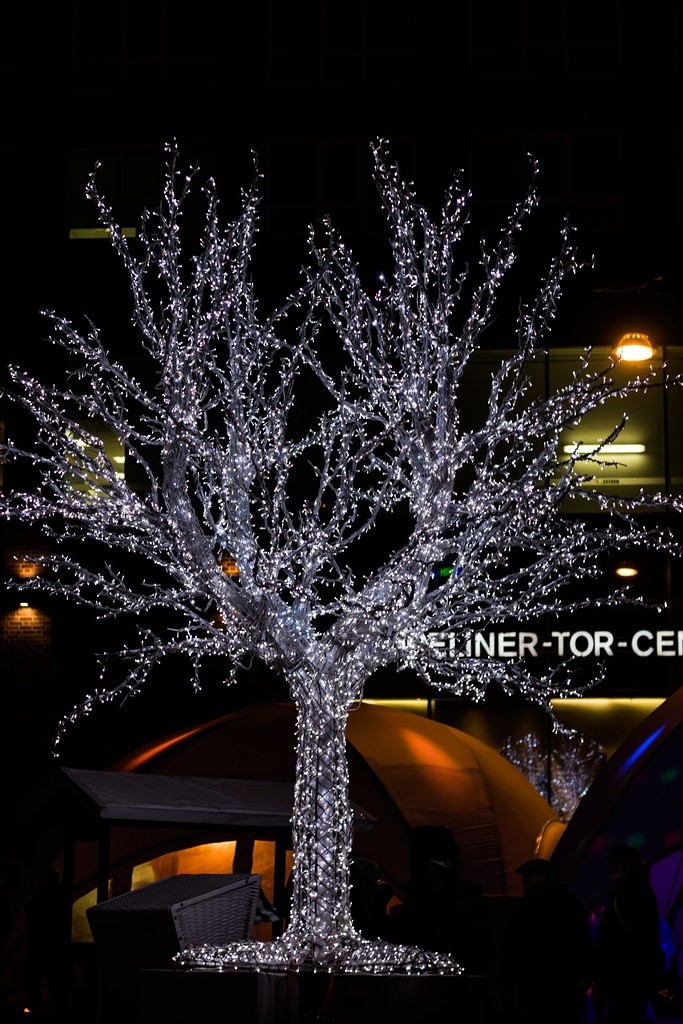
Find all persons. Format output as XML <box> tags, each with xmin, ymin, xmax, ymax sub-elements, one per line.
<box><xmin>25</xmin><ymin>865</ymin><xmax>63</xmax><ymax>1005</ymax></box>
<box><xmin>592</xmin><ymin>846</ymin><xmax>660</xmax><ymax>1024</ymax></box>
<box><xmin>402</xmin><ymin>845</ymin><xmax>478</xmax><ymax>956</ymax></box>
<box><xmin>513</xmin><ymin>859</ymin><xmax>589</xmax><ymax>1024</ymax></box>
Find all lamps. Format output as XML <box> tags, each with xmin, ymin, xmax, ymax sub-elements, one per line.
<box><xmin>562</xmin><ymin>444</ymin><xmax>646</xmax><ymax>454</ymax></box>
<box><xmin>616</xmin><ymin>332</ymin><xmax>654</xmax><ymax>362</ymax></box>
<box><xmin>615</xmin><ymin>560</ymin><xmax>638</xmax><ymax>577</ymax></box>
